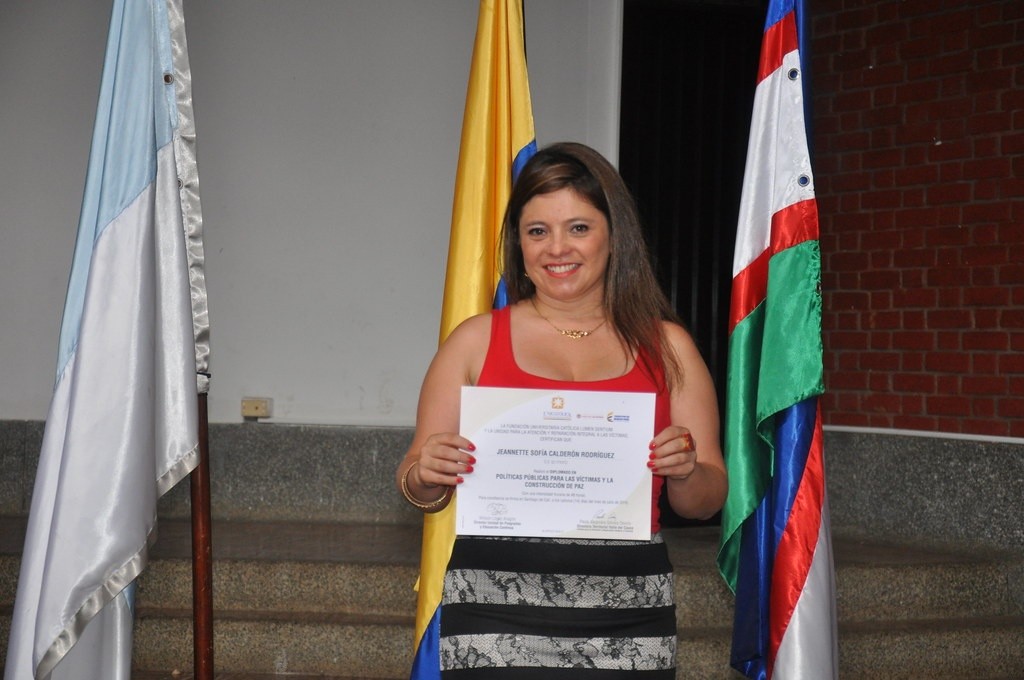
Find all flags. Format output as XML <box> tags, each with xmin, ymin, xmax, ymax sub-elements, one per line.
<box><xmin>410</xmin><ymin>0</ymin><xmax>539</xmax><ymax>680</ymax></box>
<box><xmin>716</xmin><ymin>0</ymin><xmax>837</xmax><ymax>680</ymax></box>
<box><xmin>4</xmin><ymin>0</ymin><xmax>213</xmax><ymax>680</ymax></box>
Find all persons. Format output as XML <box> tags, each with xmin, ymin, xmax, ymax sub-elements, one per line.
<box><xmin>398</xmin><ymin>144</ymin><xmax>726</xmax><ymax>680</ymax></box>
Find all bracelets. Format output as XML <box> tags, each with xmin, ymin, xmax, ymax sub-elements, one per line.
<box><xmin>402</xmin><ymin>462</ymin><xmax>449</xmax><ymax>509</ymax></box>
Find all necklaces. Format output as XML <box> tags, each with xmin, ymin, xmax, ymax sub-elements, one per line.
<box><xmin>531</xmin><ymin>297</ymin><xmax>609</xmax><ymax>340</ymax></box>
